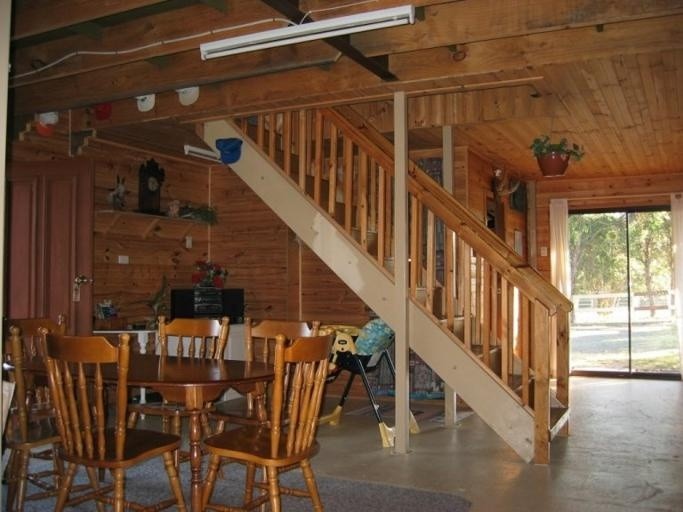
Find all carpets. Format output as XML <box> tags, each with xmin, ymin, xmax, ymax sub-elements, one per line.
<box><xmin>0</xmin><ymin>441</ymin><xmax>472</xmax><ymax>512</ymax></box>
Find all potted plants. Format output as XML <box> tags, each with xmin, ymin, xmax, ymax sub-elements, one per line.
<box><xmin>529</xmin><ymin>134</ymin><xmax>585</xmax><ymax>178</ymax></box>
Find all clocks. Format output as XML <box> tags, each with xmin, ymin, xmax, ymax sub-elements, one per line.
<box><xmin>132</xmin><ymin>157</ymin><xmax>167</xmax><ymax>216</ymax></box>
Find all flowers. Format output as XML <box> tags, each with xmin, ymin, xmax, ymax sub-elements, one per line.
<box><xmin>191</xmin><ymin>260</ymin><xmax>229</xmax><ymax>289</ymax></box>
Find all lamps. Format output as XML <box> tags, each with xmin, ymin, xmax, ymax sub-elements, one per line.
<box><xmin>200</xmin><ymin>5</ymin><xmax>417</xmax><ymax>60</ymax></box>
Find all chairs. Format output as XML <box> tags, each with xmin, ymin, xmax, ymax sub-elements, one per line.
<box><xmin>317</xmin><ymin>318</ymin><xmax>420</xmax><ymax>449</ymax></box>
<box><xmin>2</xmin><ymin>314</ymin><xmax>336</xmax><ymax>511</ymax></box>
